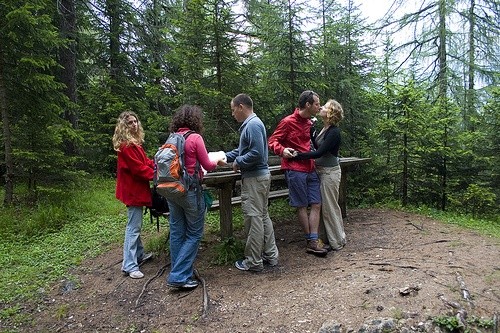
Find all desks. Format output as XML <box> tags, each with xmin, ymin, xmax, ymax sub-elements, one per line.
<box><xmin>158</xmin><ymin>155</ymin><xmax>372</xmax><ymax>240</ymax></box>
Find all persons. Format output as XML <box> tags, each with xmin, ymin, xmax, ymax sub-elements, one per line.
<box><xmin>288</xmin><ymin>99</ymin><xmax>348</xmax><ymax>250</ymax></box>
<box><xmin>165</xmin><ymin>105</ymin><xmax>228</xmax><ymax>290</ymax></box>
<box><xmin>112</xmin><ymin>111</ymin><xmax>154</xmax><ymax>279</ymax></box>
<box><xmin>268</xmin><ymin>90</ymin><xmax>331</xmax><ymax>255</ymax></box>
<box><xmin>225</xmin><ymin>94</ymin><xmax>279</xmax><ymax>271</ymax></box>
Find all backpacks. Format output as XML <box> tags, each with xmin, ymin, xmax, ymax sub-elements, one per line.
<box><xmin>152</xmin><ymin>130</ymin><xmax>201</xmax><ymax>201</ymax></box>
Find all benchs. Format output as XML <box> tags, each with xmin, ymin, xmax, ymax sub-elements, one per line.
<box><xmin>153</xmin><ymin>188</ymin><xmax>291</xmax><ymax>222</ymax></box>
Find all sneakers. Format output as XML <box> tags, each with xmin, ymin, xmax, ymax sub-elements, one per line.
<box><xmin>306</xmin><ymin>239</ymin><xmax>331</xmax><ymax>256</ymax></box>
<box><xmin>166</xmin><ymin>279</ymin><xmax>199</xmax><ymax>288</ymax></box>
<box><xmin>263</xmin><ymin>257</ymin><xmax>278</xmax><ymax>265</ymax></box>
<box><xmin>126</xmin><ymin>270</ymin><xmax>144</xmax><ymax>279</ymax></box>
<box><xmin>140</xmin><ymin>253</ymin><xmax>153</xmax><ymax>263</ymax></box>
<box><xmin>234</xmin><ymin>260</ymin><xmax>264</xmax><ymax>272</ymax></box>
